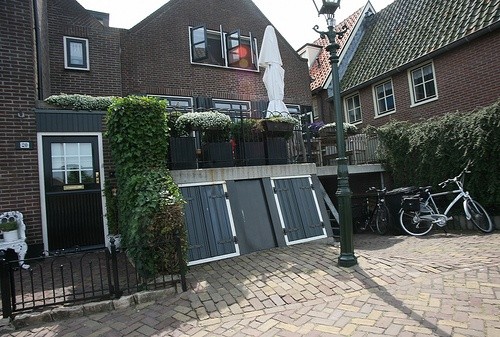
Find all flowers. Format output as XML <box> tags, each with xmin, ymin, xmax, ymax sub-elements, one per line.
<box><xmin>175</xmin><ymin>111</ymin><xmax>232</xmax><ymax>129</ymax></box>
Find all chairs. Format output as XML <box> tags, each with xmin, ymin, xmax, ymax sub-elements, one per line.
<box><xmin>0</xmin><ymin>210</ymin><xmax>31</xmax><ymax>269</ymax></box>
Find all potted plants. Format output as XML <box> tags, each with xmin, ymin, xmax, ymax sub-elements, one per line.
<box><xmin>318</xmin><ymin>122</ymin><xmax>358</xmax><ymax>136</ymax></box>
<box><xmin>106</xmin><ymin>222</ymin><xmax>123</xmax><ymax>254</ymax></box>
<box><xmin>201</xmin><ymin>130</ymin><xmax>232</xmax><ymax>168</ymax></box>
<box><xmin>0</xmin><ymin>220</ymin><xmax>18</xmax><ymax>242</ymax></box>
<box><xmin>261</xmin><ymin>116</ymin><xmax>299</xmax><ymax>133</ymax></box>
<box><xmin>232</xmin><ymin>120</ymin><xmax>264</xmax><ymax>167</ymax></box>
<box><xmin>166</xmin><ymin>111</ymin><xmax>197</xmax><ymax>171</ymax></box>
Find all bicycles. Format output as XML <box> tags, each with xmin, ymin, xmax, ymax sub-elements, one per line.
<box><xmin>358</xmin><ymin>186</ymin><xmax>391</xmax><ymax>236</ymax></box>
<box><xmin>398</xmin><ymin>158</ymin><xmax>494</xmax><ymax>237</ymax></box>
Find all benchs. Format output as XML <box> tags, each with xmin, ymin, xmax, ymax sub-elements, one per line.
<box><xmin>324</xmin><ymin>133</ymin><xmax>369</xmax><ymax>165</ymax></box>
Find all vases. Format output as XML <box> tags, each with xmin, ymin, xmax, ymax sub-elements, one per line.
<box><xmin>181</xmin><ymin>123</ymin><xmax>222</xmax><ymax>132</ymax></box>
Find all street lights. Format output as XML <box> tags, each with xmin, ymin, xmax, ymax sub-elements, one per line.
<box><xmin>311</xmin><ymin>0</ymin><xmax>359</xmax><ymax>268</ymax></box>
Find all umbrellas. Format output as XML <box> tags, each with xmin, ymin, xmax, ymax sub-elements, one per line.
<box><xmin>260</xmin><ymin>25</ymin><xmax>293</xmax><ymax>118</ymax></box>
